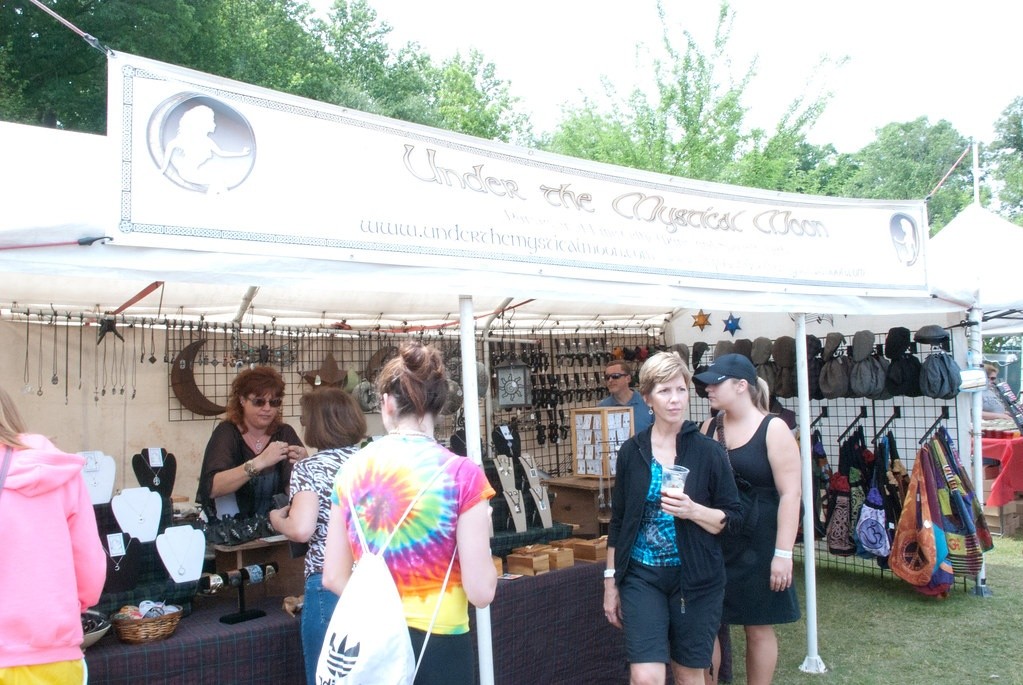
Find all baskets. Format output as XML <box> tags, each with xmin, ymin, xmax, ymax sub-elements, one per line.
<box><xmin>109</xmin><ymin>604</ymin><xmax>184</xmax><ymax>643</ymax></box>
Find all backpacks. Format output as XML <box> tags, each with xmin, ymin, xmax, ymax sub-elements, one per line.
<box><xmin>312</xmin><ymin>453</ymin><xmax>461</xmax><ymax>684</ymax></box>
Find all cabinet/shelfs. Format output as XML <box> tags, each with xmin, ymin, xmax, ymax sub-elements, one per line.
<box><xmin>540</xmin><ymin>474</ymin><xmax>615</xmax><ymax>536</ymax></box>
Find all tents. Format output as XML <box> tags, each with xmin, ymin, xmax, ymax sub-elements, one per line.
<box><xmin>0</xmin><ymin>120</ymin><xmax>985</xmax><ymax>685</ymax></box>
<box><xmin>928</xmin><ymin>200</ymin><xmax>1023</xmax><ymax>340</ymax></box>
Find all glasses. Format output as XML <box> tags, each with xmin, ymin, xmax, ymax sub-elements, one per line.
<box><xmin>605</xmin><ymin>373</ymin><xmax>629</xmax><ymax>380</ymax></box>
<box><xmin>988</xmin><ymin>377</ymin><xmax>996</xmax><ymax>381</ymax></box>
<box><xmin>239</xmin><ymin>393</ymin><xmax>282</xmax><ymax>407</ymax></box>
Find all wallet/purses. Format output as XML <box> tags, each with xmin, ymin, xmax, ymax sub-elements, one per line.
<box><xmin>271</xmin><ymin>493</ymin><xmax>309</xmax><ymax>559</ymax></box>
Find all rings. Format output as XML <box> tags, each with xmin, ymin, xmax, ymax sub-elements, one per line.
<box><xmin>276</xmin><ymin>440</ymin><xmax>280</xmax><ymax>444</ymax></box>
<box><xmin>296</xmin><ymin>454</ymin><xmax>299</xmax><ymax>459</ymax></box>
<box><xmin>782</xmin><ymin>577</ymin><xmax>786</xmax><ymax>580</ymax></box>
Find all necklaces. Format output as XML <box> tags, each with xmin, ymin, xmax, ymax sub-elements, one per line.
<box><xmin>248</xmin><ymin>432</ymin><xmax>265</xmax><ymax>450</ymax></box>
<box><xmin>164</xmin><ymin>530</ymin><xmax>195</xmax><ymax>575</ymax></box>
<box><xmin>389</xmin><ymin>430</ymin><xmax>436</xmax><ymax>441</ymax></box>
<box><xmin>85</xmin><ymin>455</ymin><xmax>101</xmax><ymax>485</ymax></box>
<box><xmin>22</xmin><ymin>308</ymin><xmax>460</xmax><ymax>409</ymax></box>
<box><xmin>140</xmin><ymin>453</ymin><xmax>169</xmax><ymax>486</ymax></box>
<box><xmin>452</xmin><ymin>426</ymin><xmax>545</xmax><ymax>512</ymax></box>
<box><xmin>120</xmin><ymin>492</ymin><xmax>151</xmax><ymax>523</ymax></box>
<box><xmin>101</xmin><ymin>538</ymin><xmax>133</xmax><ymax>572</ymax></box>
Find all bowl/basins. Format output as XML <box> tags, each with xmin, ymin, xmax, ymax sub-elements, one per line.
<box><xmin>81</xmin><ymin>610</ymin><xmax>111</xmax><ymax>652</ymax></box>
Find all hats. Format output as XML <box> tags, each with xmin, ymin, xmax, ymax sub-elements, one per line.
<box><xmin>693</xmin><ymin>353</ymin><xmax>755</xmax><ymax>385</ymax></box>
<box><xmin>438</xmin><ymin>378</ymin><xmax>462</xmax><ymax>415</ymax></box>
<box><xmin>714</xmin><ymin>325</ymin><xmax>961</xmax><ymax>400</ymax></box>
<box><xmin>352</xmin><ymin>345</ymin><xmax>400</xmax><ymax>412</ymax></box>
<box><xmin>458</xmin><ymin>361</ymin><xmax>488</xmax><ymax>398</ymax></box>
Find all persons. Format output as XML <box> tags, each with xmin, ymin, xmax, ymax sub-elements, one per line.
<box><xmin>0</xmin><ymin>385</ymin><xmax>107</xmax><ymax>685</ymax></box>
<box><xmin>269</xmin><ymin>385</ymin><xmax>367</xmax><ymax>685</ymax></box>
<box><xmin>596</xmin><ymin>360</ymin><xmax>654</xmax><ymax>435</ymax></box>
<box><xmin>970</xmin><ymin>364</ymin><xmax>1012</xmax><ymax>421</ymax></box>
<box><xmin>603</xmin><ymin>352</ymin><xmax>802</xmax><ymax>685</ymax></box>
<box><xmin>322</xmin><ymin>341</ymin><xmax>498</xmax><ymax>685</ymax></box>
<box><xmin>196</xmin><ymin>366</ymin><xmax>307</xmax><ymax>521</ymax></box>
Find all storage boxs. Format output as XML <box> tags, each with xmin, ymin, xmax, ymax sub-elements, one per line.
<box><xmin>492</xmin><ymin>537</ymin><xmax>607</xmax><ymax>577</ymax></box>
<box><xmin>971</xmin><ymin>465</ymin><xmax>1023</xmax><ymax>536</ymax></box>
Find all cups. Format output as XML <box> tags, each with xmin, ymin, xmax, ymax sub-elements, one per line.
<box><xmin>661</xmin><ymin>464</ymin><xmax>691</xmax><ymax>506</ymax></box>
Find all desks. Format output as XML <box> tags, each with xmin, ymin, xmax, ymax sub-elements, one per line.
<box><xmin>85</xmin><ymin>559</ymin><xmax>732</xmax><ymax>685</ymax></box>
<box><xmin>971</xmin><ymin>436</ymin><xmax>1023</xmax><ymax>538</ymax></box>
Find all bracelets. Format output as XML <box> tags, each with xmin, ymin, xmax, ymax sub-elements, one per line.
<box><xmin>774</xmin><ymin>548</ymin><xmax>792</xmax><ymax>558</ymax></box>
<box><xmin>244</xmin><ymin>459</ymin><xmax>260</xmax><ymax>477</ymax></box>
<box><xmin>204</xmin><ymin>564</ymin><xmax>276</xmax><ymax>595</ymax></box>
<box><xmin>604</xmin><ymin>569</ymin><xmax>615</xmax><ymax>577</ymax></box>
